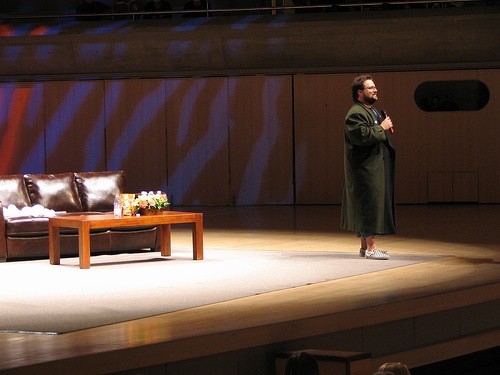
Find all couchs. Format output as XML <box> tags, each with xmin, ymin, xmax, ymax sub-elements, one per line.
<box><xmin>1</xmin><ymin>171</ymin><xmax>161</xmax><ymax>261</ymax></box>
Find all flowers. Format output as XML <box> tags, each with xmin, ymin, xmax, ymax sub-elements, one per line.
<box><xmin>134</xmin><ymin>192</ymin><xmax>171</xmax><ymax>210</ymax></box>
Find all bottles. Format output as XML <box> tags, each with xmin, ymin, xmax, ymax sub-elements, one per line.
<box><xmin>140</xmin><ymin>191</ymin><xmax>162</xmax><ymax>201</ymax></box>
<box><xmin>113</xmin><ymin>194</ymin><xmax>122</xmax><ymax>218</ymax></box>
<box><xmin>123</xmin><ymin>194</ymin><xmax>131</xmax><ymax>216</ymax></box>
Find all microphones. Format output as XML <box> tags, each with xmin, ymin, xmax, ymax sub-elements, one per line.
<box><xmin>381</xmin><ymin>109</ymin><xmax>395</xmax><ymax>134</ymax></box>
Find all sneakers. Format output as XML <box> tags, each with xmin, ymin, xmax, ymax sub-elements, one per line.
<box><xmin>360</xmin><ymin>248</ymin><xmax>387</xmax><ymax>257</ymax></box>
<box><xmin>365</xmin><ymin>250</ymin><xmax>390</xmax><ymax>259</ymax></box>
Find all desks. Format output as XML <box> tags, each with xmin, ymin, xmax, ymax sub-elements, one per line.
<box><xmin>48</xmin><ymin>213</ymin><xmax>203</xmax><ymax>268</ymax></box>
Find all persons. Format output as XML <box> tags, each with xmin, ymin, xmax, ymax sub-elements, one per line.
<box><xmin>344</xmin><ymin>76</ymin><xmax>397</xmax><ymax>260</ymax></box>
